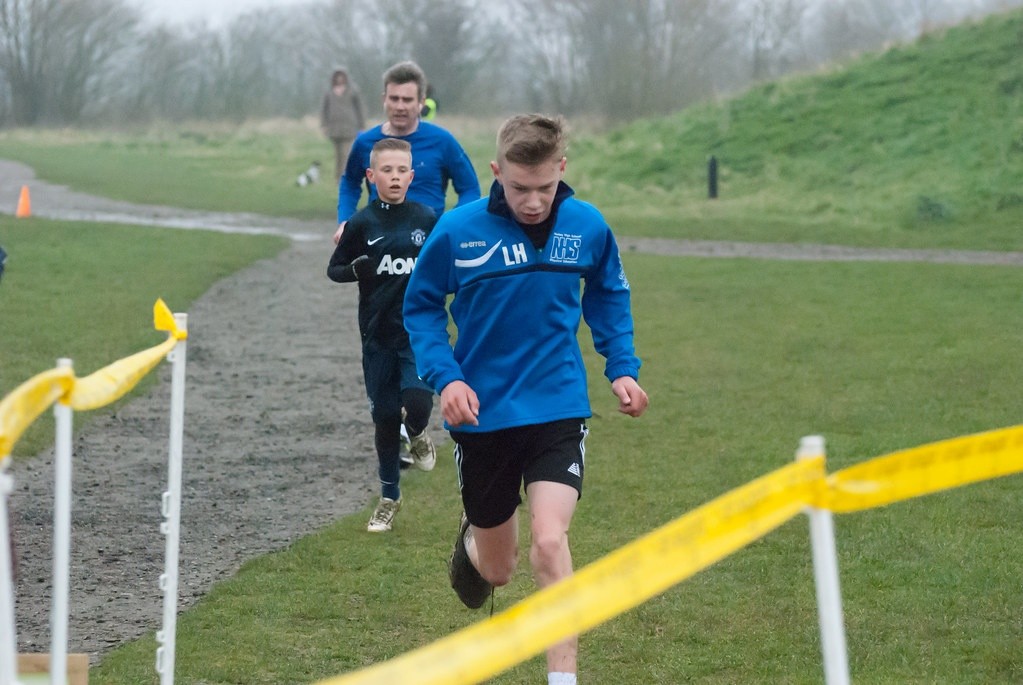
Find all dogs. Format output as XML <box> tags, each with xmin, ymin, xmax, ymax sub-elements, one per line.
<box><xmin>296</xmin><ymin>160</ymin><xmax>322</xmax><ymax>187</ymax></box>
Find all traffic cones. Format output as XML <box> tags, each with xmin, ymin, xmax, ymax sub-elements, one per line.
<box><xmin>16</xmin><ymin>185</ymin><xmax>30</xmax><ymax>218</ymax></box>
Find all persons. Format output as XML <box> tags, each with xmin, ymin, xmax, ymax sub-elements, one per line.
<box><xmin>421</xmin><ymin>85</ymin><xmax>438</xmax><ymax>121</ymax></box>
<box><xmin>334</xmin><ymin>60</ymin><xmax>482</xmax><ymax>468</ymax></box>
<box><xmin>402</xmin><ymin>112</ymin><xmax>649</xmax><ymax>685</ymax></box>
<box><xmin>320</xmin><ymin>66</ymin><xmax>366</xmax><ymax>181</ymax></box>
<box><xmin>326</xmin><ymin>138</ymin><xmax>438</xmax><ymax>532</ymax></box>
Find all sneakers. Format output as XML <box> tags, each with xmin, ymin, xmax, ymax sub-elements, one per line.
<box><xmin>404</xmin><ymin>419</ymin><xmax>436</xmax><ymax>471</ymax></box>
<box><xmin>366</xmin><ymin>486</ymin><xmax>403</xmax><ymax>535</ymax></box>
<box><xmin>447</xmin><ymin>512</ymin><xmax>494</xmax><ymax>610</ymax></box>
<box><xmin>398</xmin><ymin>432</ymin><xmax>414</xmax><ymax>466</ymax></box>
